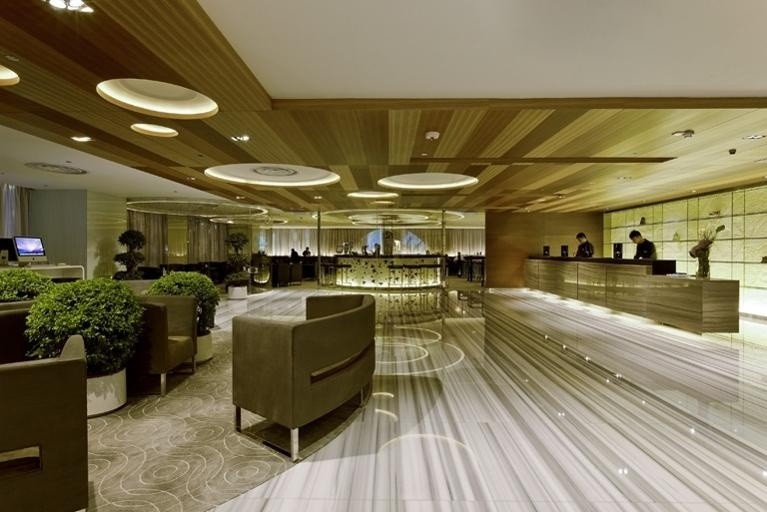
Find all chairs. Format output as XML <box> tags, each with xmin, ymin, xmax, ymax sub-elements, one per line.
<box><xmin>274</xmin><ymin>257</ymin><xmax>315</xmax><ymax>287</ymax></box>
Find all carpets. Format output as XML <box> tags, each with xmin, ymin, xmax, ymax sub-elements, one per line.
<box><xmin>87</xmin><ymin>330</ymin><xmax>373</xmax><ymax>512</ymax></box>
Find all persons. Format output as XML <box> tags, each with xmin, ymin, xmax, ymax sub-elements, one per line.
<box><xmin>629</xmin><ymin>230</ymin><xmax>657</xmax><ymax>260</ymax></box>
<box><xmin>302</xmin><ymin>247</ymin><xmax>310</xmax><ymax>256</ymax></box>
<box><xmin>290</xmin><ymin>249</ymin><xmax>298</xmax><ymax>263</ymax></box>
<box><xmin>576</xmin><ymin>232</ymin><xmax>594</xmax><ymax>259</ymax></box>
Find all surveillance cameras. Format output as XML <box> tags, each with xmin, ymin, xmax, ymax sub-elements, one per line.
<box><xmin>729</xmin><ymin>149</ymin><xmax>736</xmax><ymax>154</ymax></box>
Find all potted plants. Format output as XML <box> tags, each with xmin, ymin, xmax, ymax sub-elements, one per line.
<box><xmin>0</xmin><ymin>267</ymin><xmax>220</xmax><ymax>419</ymax></box>
<box><xmin>689</xmin><ymin>225</ymin><xmax>725</xmax><ymax>280</ymax></box>
<box><xmin>225</xmin><ymin>232</ymin><xmax>251</xmax><ymax>299</ymax></box>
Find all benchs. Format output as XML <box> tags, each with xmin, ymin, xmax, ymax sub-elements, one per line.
<box><xmin>0</xmin><ymin>334</ymin><xmax>87</xmax><ymax>512</ymax></box>
<box><xmin>232</xmin><ymin>294</ymin><xmax>375</xmax><ymax>462</ymax></box>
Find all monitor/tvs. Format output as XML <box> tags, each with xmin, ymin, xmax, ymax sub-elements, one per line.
<box><xmin>543</xmin><ymin>245</ymin><xmax>550</xmax><ymax>256</ymax></box>
<box><xmin>12</xmin><ymin>235</ymin><xmax>49</xmax><ymax>266</ymax></box>
<box><xmin>613</xmin><ymin>243</ymin><xmax>622</xmax><ymax>259</ymax></box>
<box><xmin>560</xmin><ymin>245</ymin><xmax>568</xmax><ymax>256</ymax></box>
<box><xmin>0</xmin><ymin>238</ymin><xmax>18</xmax><ymax>266</ymax></box>
